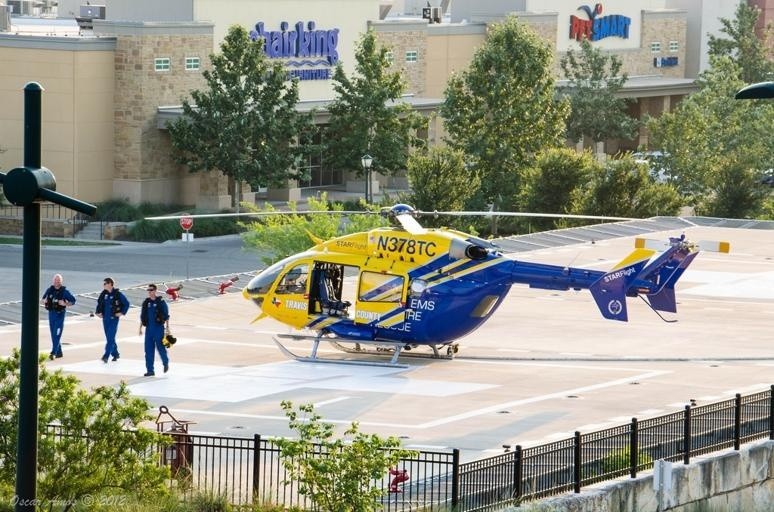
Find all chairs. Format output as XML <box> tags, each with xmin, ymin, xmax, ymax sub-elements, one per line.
<box><xmin>319</xmin><ymin>271</ymin><xmax>351</xmax><ymax>315</ymax></box>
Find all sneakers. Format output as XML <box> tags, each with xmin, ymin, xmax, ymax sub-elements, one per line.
<box><xmin>164</xmin><ymin>364</ymin><xmax>168</xmax><ymax>372</ymax></box>
<box><xmin>48</xmin><ymin>354</ymin><xmax>62</xmax><ymax>360</ymax></box>
<box><xmin>102</xmin><ymin>355</ymin><xmax>119</xmax><ymax>362</ymax></box>
<box><xmin>144</xmin><ymin>372</ymin><xmax>155</xmax><ymax>376</ymax></box>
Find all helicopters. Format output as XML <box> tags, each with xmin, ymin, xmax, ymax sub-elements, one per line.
<box><xmin>145</xmin><ymin>204</ymin><xmax>729</xmax><ymax>369</ymax></box>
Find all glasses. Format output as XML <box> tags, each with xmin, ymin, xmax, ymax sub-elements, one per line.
<box><xmin>104</xmin><ymin>283</ymin><xmax>108</xmax><ymax>285</ymax></box>
<box><xmin>147</xmin><ymin>289</ymin><xmax>154</xmax><ymax>291</ymax></box>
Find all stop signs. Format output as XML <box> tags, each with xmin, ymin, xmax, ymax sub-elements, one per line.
<box><xmin>180</xmin><ymin>213</ymin><xmax>193</xmax><ymax>231</ymax></box>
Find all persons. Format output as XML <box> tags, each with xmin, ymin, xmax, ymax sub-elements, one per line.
<box><xmin>41</xmin><ymin>274</ymin><xmax>76</xmax><ymax>359</ymax></box>
<box><xmin>95</xmin><ymin>278</ymin><xmax>129</xmax><ymax>363</ymax></box>
<box><xmin>139</xmin><ymin>285</ymin><xmax>169</xmax><ymax>376</ymax></box>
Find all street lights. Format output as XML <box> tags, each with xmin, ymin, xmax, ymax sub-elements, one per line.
<box><xmin>360</xmin><ymin>153</ymin><xmax>373</xmax><ymax>203</ymax></box>
